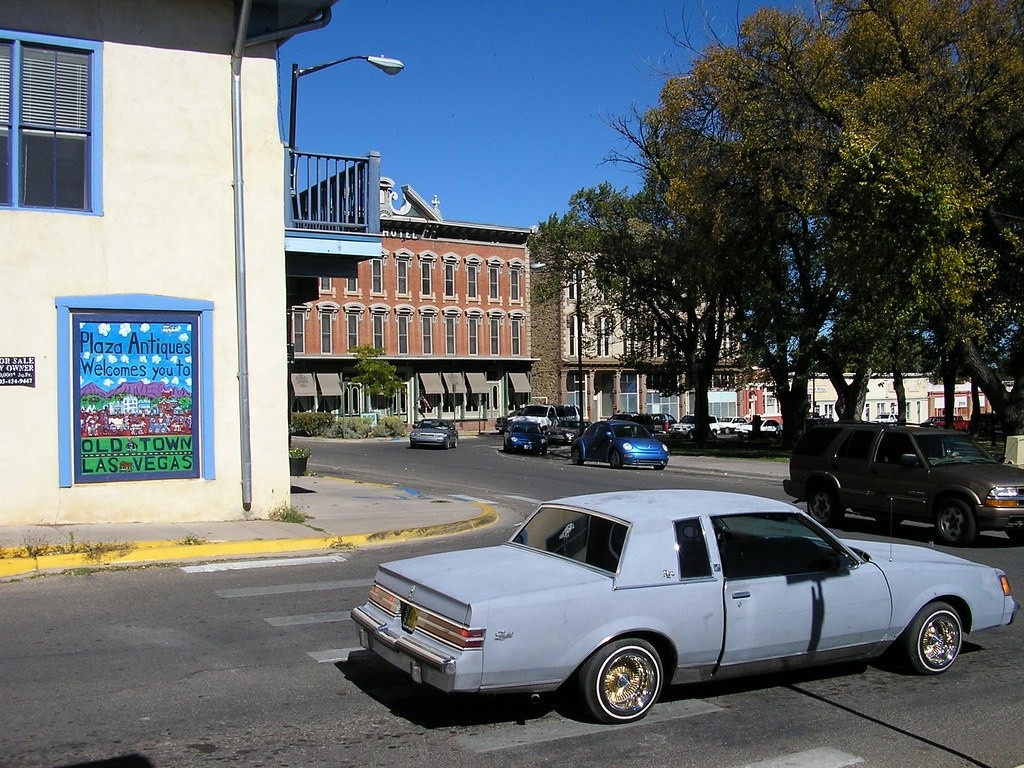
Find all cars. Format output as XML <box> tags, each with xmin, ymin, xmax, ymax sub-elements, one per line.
<box><xmin>407</xmin><ymin>419</ymin><xmax>459</xmax><ymax>450</ymax></box>
<box><xmin>571</xmin><ymin>420</ymin><xmax>670</xmax><ymax>469</ymax></box>
<box><xmin>874</xmin><ymin>413</ymin><xmax>900</xmax><ymax>425</ymax></box>
<box><xmin>735</xmin><ymin>418</ymin><xmax>784</xmax><ymax>439</ymax></box>
<box><xmin>925</xmin><ymin>416</ymin><xmax>969</xmax><ymax>432</ymax></box>
<box><xmin>642</xmin><ymin>413</ymin><xmax>676</xmax><ymax>433</ymax></box>
<box><xmin>718</xmin><ymin>416</ymin><xmax>748</xmax><ymax>434</ymax></box>
<box><xmin>350</xmin><ymin>489</ymin><xmax>1022</xmax><ymax>725</ymax></box>
<box><xmin>502</xmin><ymin>420</ymin><xmax>549</xmax><ymax>457</ymax></box>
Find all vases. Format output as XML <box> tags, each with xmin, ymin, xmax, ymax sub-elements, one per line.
<box><xmin>289</xmin><ymin>457</ymin><xmax>308</xmax><ymax>477</ymax></box>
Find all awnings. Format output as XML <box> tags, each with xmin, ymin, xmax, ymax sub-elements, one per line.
<box><xmin>316</xmin><ymin>373</ymin><xmax>344</xmax><ymax>396</ymax></box>
<box><xmin>290</xmin><ymin>373</ymin><xmax>318</xmax><ymax>396</ymax></box>
<box><xmin>419</xmin><ymin>371</ymin><xmax>490</xmax><ymax>394</ymax></box>
<box><xmin>509</xmin><ymin>372</ymin><xmax>532</xmax><ymax>393</ymax></box>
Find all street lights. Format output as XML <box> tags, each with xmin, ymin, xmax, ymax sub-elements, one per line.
<box><xmin>530</xmin><ymin>261</ymin><xmax>585</xmax><ymax>437</ymax></box>
<box><xmin>290</xmin><ymin>54</ymin><xmax>413</xmax><ymax>218</ymax></box>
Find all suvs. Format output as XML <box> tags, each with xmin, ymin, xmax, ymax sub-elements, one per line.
<box><xmin>671</xmin><ymin>413</ymin><xmax>721</xmax><ymax>439</ymax></box>
<box><xmin>496</xmin><ymin>405</ymin><xmax>581</xmax><ymax>432</ymax></box>
<box><xmin>607</xmin><ymin>410</ymin><xmax>654</xmax><ymax>435</ymax></box>
<box><xmin>781</xmin><ymin>415</ymin><xmax>1024</xmax><ymax>547</ymax></box>
<box><xmin>508</xmin><ymin>403</ymin><xmax>558</xmax><ymax>436</ymax></box>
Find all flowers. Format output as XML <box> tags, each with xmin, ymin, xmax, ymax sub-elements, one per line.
<box><xmin>289</xmin><ymin>441</ymin><xmax>313</xmax><ymax>458</ymax></box>
